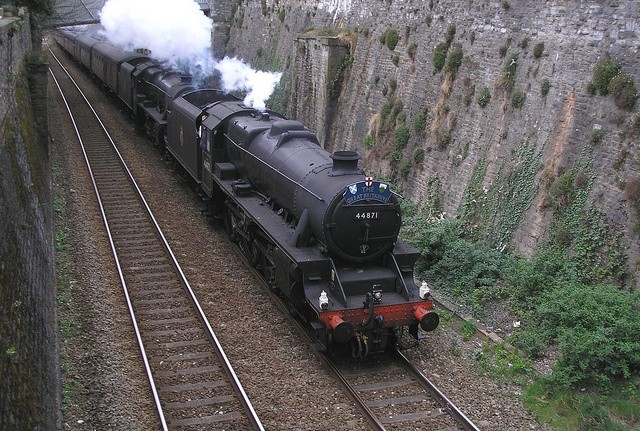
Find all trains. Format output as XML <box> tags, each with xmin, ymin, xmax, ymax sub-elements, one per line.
<box><xmin>50</xmin><ymin>26</ymin><xmax>439</xmax><ymax>363</ymax></box>
